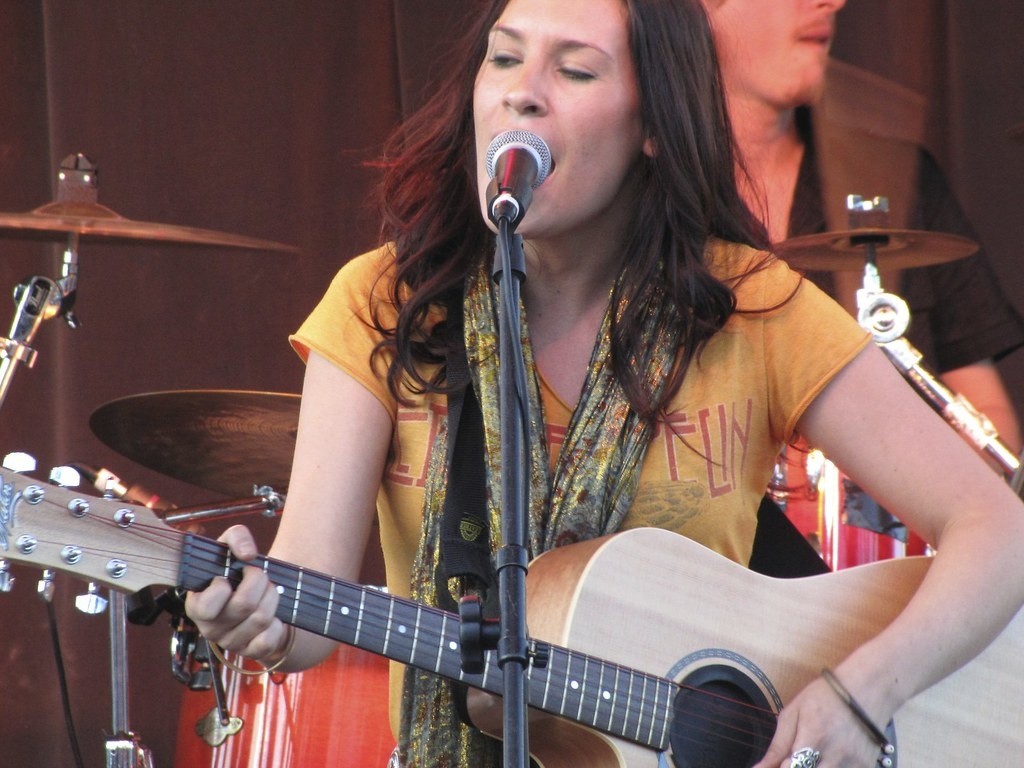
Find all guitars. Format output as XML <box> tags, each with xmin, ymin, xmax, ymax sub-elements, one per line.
<box><xmin>0</xmin><ymin>447</ymin><xmax>1024</xmax><ymax>767</ymax></box>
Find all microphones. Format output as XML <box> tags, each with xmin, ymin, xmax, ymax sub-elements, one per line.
<box><xmin>486</xmin><ymin>128</ymin><xmax>551</xmax><ymax>222</ymax></box>
<box><xmin>72</xmin><ymin>459</ymin><xmax>206</xmax><ymax>536</ymax></box>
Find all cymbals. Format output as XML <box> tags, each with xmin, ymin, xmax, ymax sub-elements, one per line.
<box><xmin>85</xmin><ymin>387</ymin><xmax>301</xmax><ymax>498</ymax></box>
<box><xmin>775</xmin><ymin>227</ymin><xmax>982</xmax><ymax>273</ymax></box>
<box><xmin>2</xmin><ymin>197</ymin><xmax>302</xmax><ymax>258</ymax></box>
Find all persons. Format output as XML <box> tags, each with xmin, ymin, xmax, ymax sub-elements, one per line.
<box><xmin>183</xmin><ymin>0</ymin><xmax>1024</xmax><ymax>768</ymax></box>
<box><xmin>702</xmin><ymin>0</ymin><xmax>1021</xmax><ymax>487</ymax></box>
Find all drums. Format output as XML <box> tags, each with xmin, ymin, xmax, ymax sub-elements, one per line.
<box><xmin>169</xmin><ymin>574</ymin><xmax>395</xmax><ymax>768</ymax></box>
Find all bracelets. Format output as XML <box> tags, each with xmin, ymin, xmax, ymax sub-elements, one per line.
<box><xmin>822</xmin><ymin>667</ymin><xmax>894</xmax><ymax>768</ymax></box>
<box><xmin>209</xmin><ymin>625</ymin><xmax>296</xmax><ymax>675</ymax></box>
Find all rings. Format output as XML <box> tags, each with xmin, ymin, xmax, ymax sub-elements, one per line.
<box><xmin>790</xmin><ymin>748</ymin><xmax>821</xmax><ymax>768</ymax></box>
